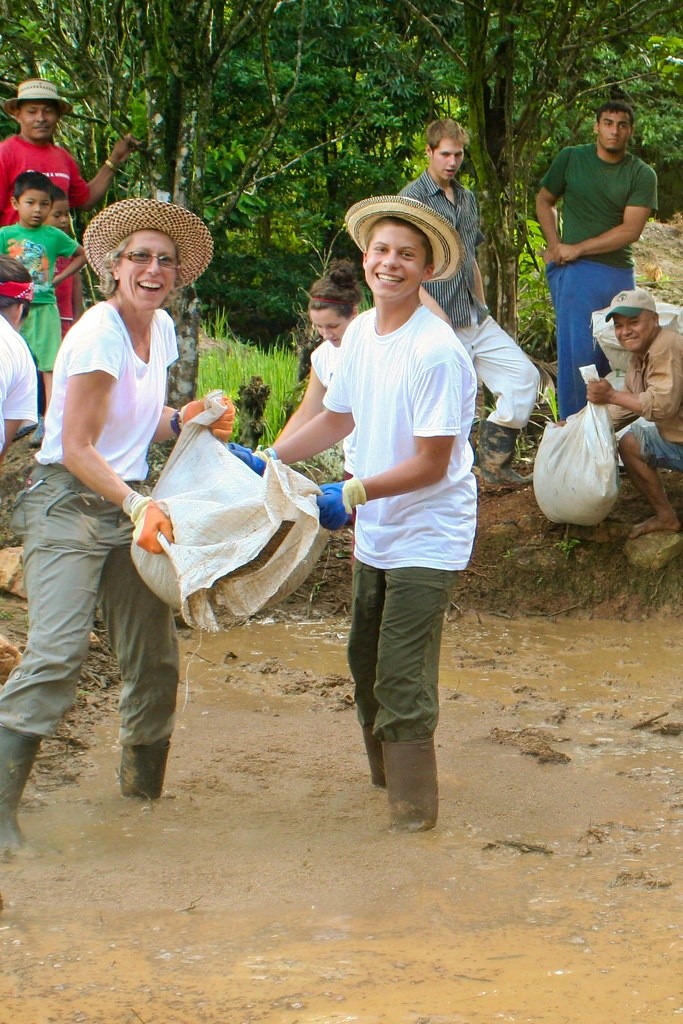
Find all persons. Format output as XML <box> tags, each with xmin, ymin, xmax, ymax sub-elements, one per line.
<box><xmin>0</xmin><ymin>172</ymin><xmax>88</xmax><ymax>447</ymax></box>
<box><xmin>0</xmin><ymin>256</ymin><xmax>38</xmax><ymax>462</ymax></box>
<box><xmin>230</xmin><ymin>197</ymin><xmax>479</xmax><ymax>833</ymax></box>
<box><xmin>554</xmin><ymin>290</ymin><xmax>683</xmax><ymax>539</ymax></box>
<box><xmin>536</xmin><ymin>102</ymin><xmax>658</xmax><ymax>474</ymax></box>
<box><xmin>275</xmin><ymin>261</ymin><xmax>362</xmax><ymax>564</ymax></box>
<box><xmin>0</xmin><ymin>78</ymin><xmax>141</xmax><ymax>226</ymax></box>
<box><xmin>0</xmin><ymin>198</ymin><xmax>236</xmax><ymax>855</ymax></box>
<box><xmin>396</xmin><ymin>119</ymin><xmax>541</xmax><ymax>492</ymax></box>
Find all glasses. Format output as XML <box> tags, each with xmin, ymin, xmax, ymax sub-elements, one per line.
<box><xmin>119</xmin><ymin>252</ymin><xmax>179</xmax><ymax>268</ymax></box>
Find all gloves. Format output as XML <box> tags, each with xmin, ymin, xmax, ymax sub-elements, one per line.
<box><xmin>121</xmin><ymin>492</ymin><xmax>174</xmax><ymax>554</ymax></box>
<box><xmin>180</xmin><ymin>396</ymin><xmax>235</xmax><ymax>443</ymax></box>
<box><xmin>227</xmin><ymin>442</ymin><xmax>277</xmax><ymax>477</ymax></box>
<box><xmin>317</xmin><ymin>476</ymin><xmax>367</xmax><ymax>532</ymax></box>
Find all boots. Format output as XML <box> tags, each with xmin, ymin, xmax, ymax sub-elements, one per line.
<box><xmin>0</xmin><ymin>726</ymin><xmax>45</xmax><ymax>862</ymax></box>
<box><xmin>380</xmin><ymin>739</ymin><xmax>439</xmax><ymax>836</ymax></box>
<box><xmin>479</xmin><ymin>419</ymin><xmax>533</xmax><ymax>494</ymax></box>
<box><xmin>363</xmin><ymin>730</ymin><xmax>388</xmax><ymax>790</ymax></box>
<box><xmin>119</xmin><ymin>742</ymin><xmax>170</xmax><ymax>802</ymax></box>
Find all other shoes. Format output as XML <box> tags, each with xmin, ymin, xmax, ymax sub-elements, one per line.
<box><xmin>12</xmin><ymin>425</ymin><xmax>39</xmax><ymax>439</ymax></box>
<box><xmin>29</xmin><ymin>418</ymin><xmax>46</xmax><ymax>445</ymax></box>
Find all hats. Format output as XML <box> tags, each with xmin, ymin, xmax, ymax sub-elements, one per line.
<box><xmin>345</xmin><ymin>195</ymin><xmax>462</xmax><ymax>283</ymax></box>
<box><xmin>4</xmin><ymin>79</ymin><xmax>73</xmax><ymax>116</ymax></box>
<box><xmin>605</xmin><ymin>290</ymin><xmax>657</xmax><ymax>322</ymax></box>
<box><xmin>83</xmin><ymin>199</ymin><xmax>213</xmax><ymax>289</ymax></box>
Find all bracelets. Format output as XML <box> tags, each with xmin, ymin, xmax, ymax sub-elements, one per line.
<box><xmin>171</xmin><ymin>410</ymin><xmax>182</xmax><ymax>439</ymax></box>
<box><xmin>264</xmin><ymin>448</ymin><xmax>278</xmax><ymax>460</ymax></box>
<box><xmin>105</xmin><ymin>160</ymin><xmax>118</xmax><ymax>173</ymax></box>
